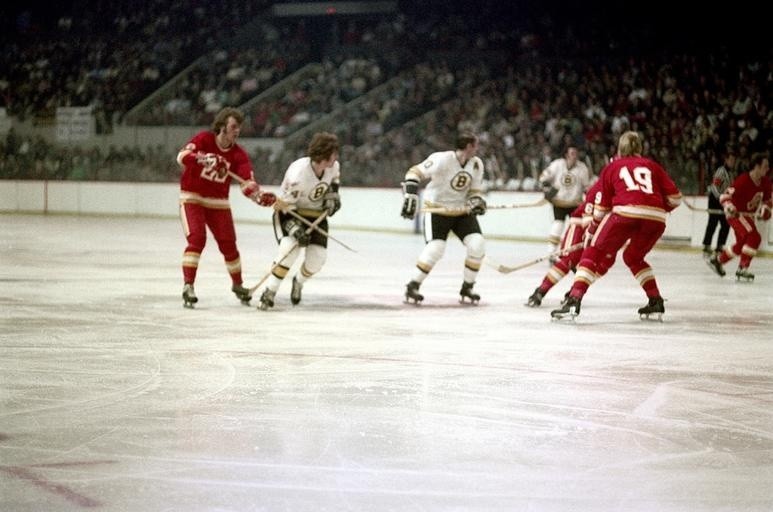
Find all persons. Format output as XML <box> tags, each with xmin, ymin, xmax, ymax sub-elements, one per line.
<box><xmin>527</xmin><ymin>180</ymin><xmax>601</xmax><ymax>308</ymax></box>
<box><xmin>537</xmin><ymin>145</ymin><xmax>592</xmax><ymax>268</ymax></box>
<box><xmin>259</xmin><ymin>132</ymin><xmax>340</xmax><ymax>307</ymax></box>
<box><xmin>701</xmin><ymin>151</ymin><xmax>735</xmax><ymax>254</ymax></box>
<box><xmin>549</xmin><ymin>130</ymin><xmax>681</xmax><ymax>318</ymax></box>
<box><xmin>176</xmin><ymin>107</ymin><xmax>275</xmax><ymax>304</ymax></box>
<box><xmin>710</xmin><ymin>154</ymin><xmax>771</xmax><ymax>279</ymax></box>
<box><xmin>399</xmin><ymin>131</ymin><xmax>485</xmax><ymax>300</ymax></box>
<box><xmin>1</xmin><ymin>1</ymin><xmax>771</xmax><ymax>195</ymax></box>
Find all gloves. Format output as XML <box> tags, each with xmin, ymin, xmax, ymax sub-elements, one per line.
<box><xmin>723</xmin><ymin>203</ymin><xmax>738</xmax><ymax>219</ymax></box>
<box><xmin>760</xmin><ymin>201</ymin><xmax>771</xmax><ymax>218</ymax></box>
<box><xmin>542</xmin><ymin>182</ymin><xmax>557</xmax><ymax>200</ymax></box>
<box><xmin>468</xmin><ymin>198</ymin><xmax>486</xmax><ymax>216</ymax></box>
<box><xmin>203</xmin><ymin>154</ymin><xmax>231</xmax><ymax>181</ymax></box>
<box><xmin>255</xmin><ymin>191</ymin><xmax>276</xmax><ymax>205</ymax></box>
<box><xmin>401</xmin><ymin>195</ymin><xmax>417</xmax><ymax>220</ymax></box>
<box><xmin>321</xmin><ymin>192</ymin><xmax>341</xmax><ymax>215</ymax></box>
<box><xmin>582</xmin><ymin>221</ymin><xmax>599</xmax><ymax>247</ymax></box>
<box><xmin>289</xmin><ymin>225</ymin><xmax>311</xmax><ymax>247</ymax></box>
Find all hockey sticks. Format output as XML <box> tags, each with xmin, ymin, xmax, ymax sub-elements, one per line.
<box><xmin>419</xmin><ymin>181</ymin><xmax>559</xmax><ymax>213</ymax></box>
<box><xmin>231</xmin><ymin>210</ymin><xmax>328</xmax><ymax>297</ymax></box>
<box><xmin>226</xmin><ymin>169</ymin><xmax>366</xmax><ymax>251</ymax></box>
<box><xmin>680</xmin><ymin>192</ymin><xmax>759</xmax><ymax>219</ymax></box>
<box><xmin>496</xmin><ymin>234</ymin><xmax>582</xmax><ymax>273</ymax></box>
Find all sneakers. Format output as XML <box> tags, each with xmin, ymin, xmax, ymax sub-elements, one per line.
<box><xmin>407</xmin><ymin>282</ymin><xmax>424</xmax><ymax>301</ymax></box>
<box><xmin>551</xmin><ymin>298</ymin><xmax>580</xmax><ymax>317</ymax></box>
<box><xmin>460</xmin><ymin>282</ymin><xmax>480</xmax><ymax>300</ymax></box>
<box><xmin>640</xmin><ymin>297</ymin><xmax>665</xmax><ymax>312</ymax></box>
<box><xmin>182</xmin><ymin>282</ymin><xmax>198</xmax><ymax>302</ymax></box>
<box><xmin>532</xmin><ymin>287</ymin><xmax>544</xmax><ymax>305</ymax></box>
<box><xmin>735</xmin><ymin>266</ymin><xmax>755</xmax><ymax>278</ymax></box>
<box><xmin>710</xmin><ymin>254</ymin><xmax>726</xmax><ymax>276</ymax></box>
<box><xmin>233</xmin><ymin>279</ymin><xmax>303</xmax><ymax>308</ymax></box>
